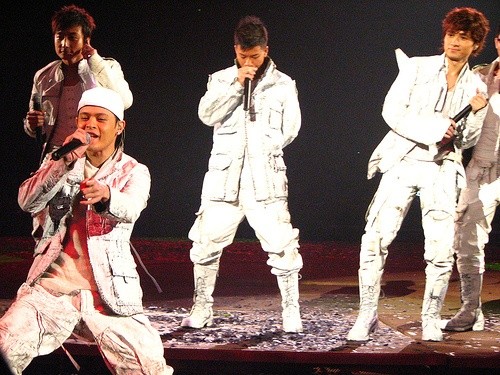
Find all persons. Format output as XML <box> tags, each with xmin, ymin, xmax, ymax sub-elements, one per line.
<box><xmin>0</xmin><ymin>86</ymin><xmax>175</xmax><ymax>375</ymax></box>
<box><xmin>181</xmin><ymin>15</ymin><xmax>304</xmax><ymax>333</ymax></box>
<box><xmin>23</xmin><ymin>4</ymin><xmax>133</xmax><ymax>242</ymax></box>
<box><xmin>347</xmin><ymin>9</ymin><xmax>490</xmax><ymax>347</ymax></box>
<box><xmin>444</xmin><ymin>30</ymin><xmax>500</xmax><ymax>331</ymax></box>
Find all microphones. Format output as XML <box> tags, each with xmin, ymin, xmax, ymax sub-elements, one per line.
<box><xmin>451</xmin><ymin>92</ymin><xmax>489</xmax><ymax>126</ymax></box>
<box><xmin>243</xmin><ymin>77</ymin><xmax>252</xmax><ymax>111</ymax></box>
<box><xmin>52</xmin><ymin>133</ymin><xmax>92</xmax><ymax>161</ymax></box>
<box><xmin>32</xmin><ymin>93</ymin><xmax>44</xmax><ymax>144</ymax></box>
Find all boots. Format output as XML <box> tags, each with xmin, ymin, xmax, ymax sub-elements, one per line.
<box><xmin>275</xmin><ymin>271</ymin><xmax>304</xmax><ymax>334</ymax></box>
<box><xmin>181</xmin><ymin>264</ymin><xmax>218</xmax><ymax>329</ymax></box>
<box><xmin>346</xmin><ymin>268</ymin><xmax>382</xmax><ymax>341</ymax></box>
<box><xmin>421</xmin><ymin>278</ymin><xmax>450</xmax><ymax>341</ymax></box>
<box><xmin>439</xmin><ymin>273</ymin><xmax>485</xmax><ymax>332</ymax></box>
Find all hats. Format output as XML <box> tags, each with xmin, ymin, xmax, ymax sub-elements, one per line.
<box><xmin>77</xmin><ymin>86</ymin><xmax>125</xmax><ymax>121</ymax></box>
